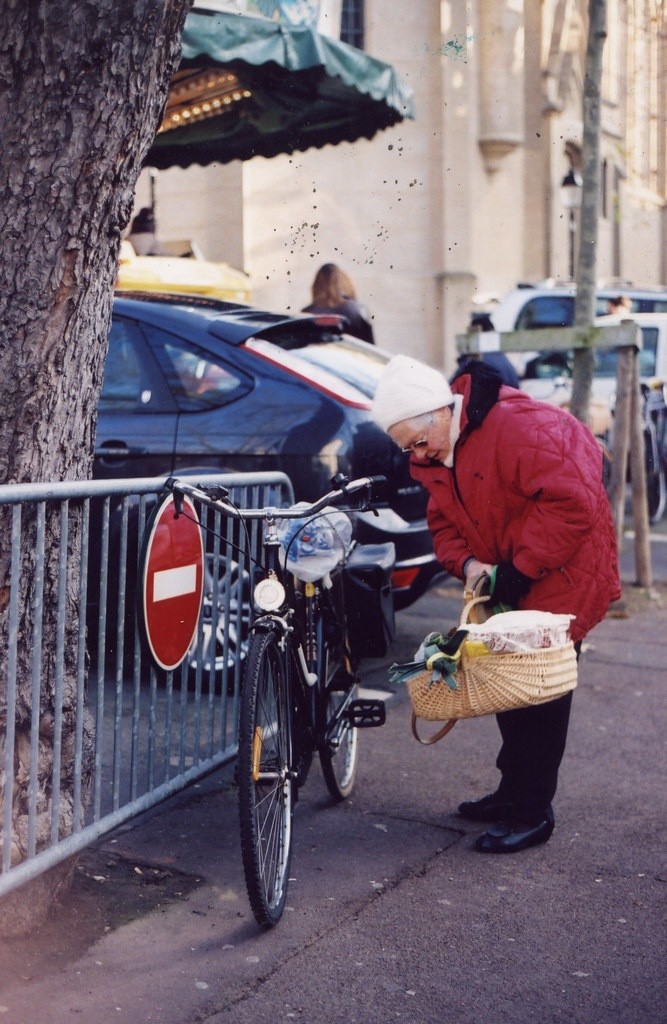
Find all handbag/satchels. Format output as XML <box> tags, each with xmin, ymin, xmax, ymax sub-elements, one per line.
<box><xmin>342</xmin><ymin>542</ymin><xmax>396</xmax><ymax>658</ymax></box>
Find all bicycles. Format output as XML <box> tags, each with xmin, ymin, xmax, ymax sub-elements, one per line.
<box><xmin>142</xmin><ymin>470</ymin><xmax>395</xmax><ymax>931</ymax></box>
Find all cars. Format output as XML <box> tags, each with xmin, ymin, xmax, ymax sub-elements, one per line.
<box><xmin>86</xmin><ymin>284</ymin><xmax>441</xmax><ymax>667</ymax></box>
<box><xmin>480</xmin><ymin>283</ymin><xmax>667</xmax><ymax>524</ymax></box>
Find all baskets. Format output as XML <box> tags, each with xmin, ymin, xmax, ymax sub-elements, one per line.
<box><xmin>406</xmin><ymin>598</ymin><xmax>578</xmax><ymax>715</ymax></box>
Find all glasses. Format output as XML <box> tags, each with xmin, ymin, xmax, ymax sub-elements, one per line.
<box><xmin>402</xmin><ymin>412</ymin><xmax>437</xmax><ymax>454</ymax></box>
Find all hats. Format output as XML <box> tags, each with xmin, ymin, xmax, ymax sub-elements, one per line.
<box><xmin>374</xmin><ymin>354</ymin><xmax>452</xmax><ymax>432</ymax></box>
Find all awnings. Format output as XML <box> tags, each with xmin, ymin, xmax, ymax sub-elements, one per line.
<box><xmin>142</xmin><ymin>19</ymin><xmax>417</xmax><ymax>170</ymax></box>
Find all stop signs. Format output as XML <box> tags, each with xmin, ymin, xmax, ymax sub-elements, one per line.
<box><xmin>144</xmin><ymin>493</ymin><xmax>206</xmax><ymax>668</ymax></box>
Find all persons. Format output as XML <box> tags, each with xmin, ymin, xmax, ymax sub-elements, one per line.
<box><xmin>302</xmin><ymin>263</ymin><xmax>374</xmax><ymax>344</ymax></box>
<box><xmin>372</xmin><ymin>356</ymin><xmax>622</xmax><ymax>854</ymax></box>
<box><xmin>453</xmin><ymin>313</ymin><xmax>518</xmax><ymax>389</ymax></box>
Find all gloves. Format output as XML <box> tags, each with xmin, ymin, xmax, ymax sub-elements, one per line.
<box><xmin>485</xmin><ymin>561</ymin><xmax>534</xmax><ymax>611</ymax></box>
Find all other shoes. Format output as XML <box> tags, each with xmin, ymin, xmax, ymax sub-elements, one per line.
<box><xmin>474</xmin><ymin>816</ymin><xmax>555</xmax><ymax>851</ymax></box>
<box><xmin>459</xmin><ymin>796</ymin><xmax>509</xmax><ymax>816</ymax></box>
